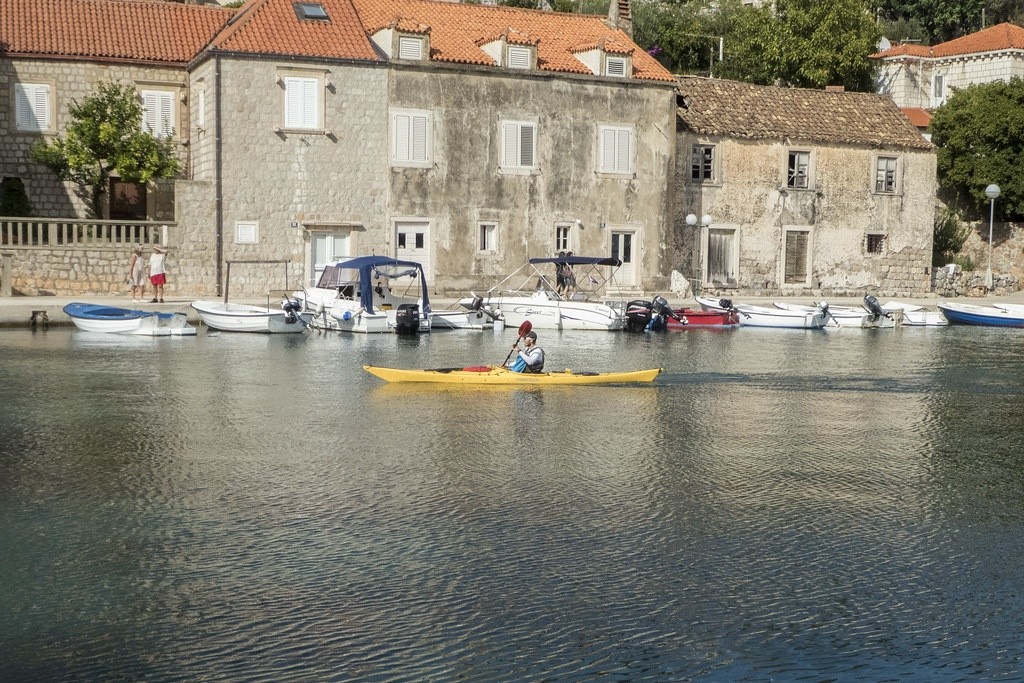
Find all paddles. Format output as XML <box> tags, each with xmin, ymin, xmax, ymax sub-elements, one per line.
<box><xmin>500</xmin><ymin>320</ymin><xmax>532</xmax><ymax>368</ymax></box>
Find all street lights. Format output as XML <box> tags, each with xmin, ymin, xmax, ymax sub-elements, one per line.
<box><xmin>685</xmin><ymin>213</ymin><xmax>714</xmax><ymax>293</ymax></box>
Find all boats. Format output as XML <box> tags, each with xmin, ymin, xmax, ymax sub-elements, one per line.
<box><xmin>281</xmin><ymin>256</ymin><xmax>433</xmax><ymax>334</ymax></box>
<box><xmin>430</xmin><ymin>309</ymin><xmax>488</xmax><ymax>328</ymax></box>
<box><xmin>937</xmin><ymin>302</ymin><xmax>1024</xmax><ymax>328</ymax></box>
<box><xmin>772</xmin><ymin>301</ymin><xmax>950</xmax><ymax>327</ymax></box>
<box><xmin>985</xmin><ymin>184</ymin><xmax>1001</xmax><ymax>293</ymax></box>
<box><xmin>694</xmin><ymin>295</ymin><xmax>832</xmax><ymax>328</ymax></box>
<box><xmin>362</xmin><ymin>364</ymin><xmax>661</xmax><ymax>383</ymax></box>
<box><xmin>458</xmin><ymin>256</ymin><xmax>628</xmax><ymax>331</ymax></box>
<box><xmin>63</xmin><ymin>302</ymin><xmax>198</xmax><ymax>337</ymax></box>
<box><xmin>993</xmin><ymin>302</ymin><xmax>1024</xmax><ymax>312</ymax></box>
<box><xmin>191</xmin><ymin>300</ymin><xmax>315</xmax><ymax>333</ymax></box>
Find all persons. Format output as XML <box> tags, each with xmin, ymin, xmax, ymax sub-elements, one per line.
<box><xmin>563</xmin><ymin>252</ymin><xmax>577</xmax><ymax>300</ymax></box>
<box><xmin>129</xmin><ymin>244</ymin><xmax>149</xmax><ymax>303</ymax></box>
<box><xmin>500</xmin><ymin>331</ymin><xmax>544</xmax><ymax>373</ymax></box>
<box><xmin>555</xmin><ymin>252</ymin><xmax>569</xmax><ymax>299</ymax></box>
<box><xmin>148</xmin><ymin>243</ymin><xmax>167</xmax><ymax>303</ymax></box>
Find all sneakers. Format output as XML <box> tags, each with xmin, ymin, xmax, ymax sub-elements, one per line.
<box><xmin>132</xmin><ymin>300</ymin><xmax>136</xmax><ymax>303</ymax></box>
<box><xmin>150</xmin><ymin>298</ymin><xmax>157</xmax><ymax>302</ymax></box>
<box><xmin>140</xmin><ymin>300</ymin><xmax>148</xmax><ymax>303</ymax></box>
<box><xmin>159</xmin><ymin>299</ymin><xmax>164</xmax><ymax>303</ymax></box>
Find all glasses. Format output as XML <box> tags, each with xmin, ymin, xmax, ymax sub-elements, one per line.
<box><xmin>524</xmin><ymin>336</ymin><xmax>535</xmax><ymax>340</ymax></box>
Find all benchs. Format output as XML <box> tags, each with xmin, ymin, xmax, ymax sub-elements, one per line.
<box><xmin>228</xmin><ymin>310</ymin><xmax>251</xmax><ymax>313</ymax></box>
